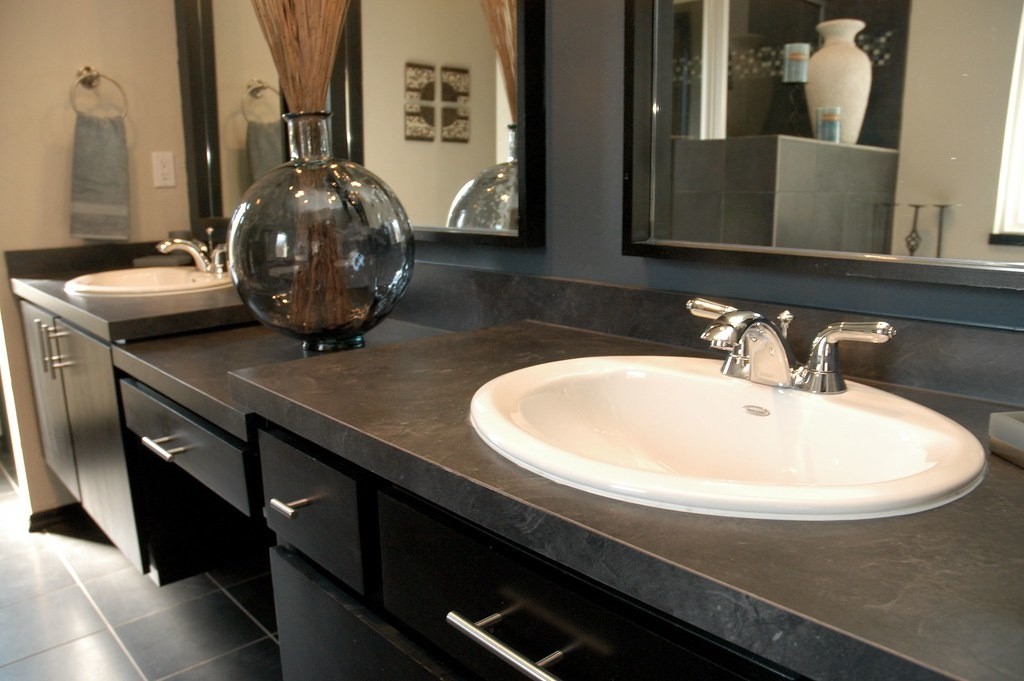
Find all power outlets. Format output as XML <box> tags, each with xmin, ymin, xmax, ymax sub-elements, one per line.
<box><xmin>149</xmin><ymin>150</ymin><xmax>178</xmax><ymax>189</ymax></box>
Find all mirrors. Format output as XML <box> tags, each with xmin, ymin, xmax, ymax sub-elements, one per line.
<box><xmin>622</xmin><ymin>0</ymin><xmax>1024</xmax><ymax>293</ymax></box>
<box><xmin>172</xmin><ymin>0</ymin><xmax>546</xmax><ymax>253</ymax></box>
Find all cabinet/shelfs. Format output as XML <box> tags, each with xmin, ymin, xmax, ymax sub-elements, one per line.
<box><xmin>13</xmin><ymin>266</ymin><xmax>263</xmax><ymax>586</ymax></box>
<box><xmin>223</xmin><ymin>315</ymin><xmax>1024</xmax><ymax>681</ymax></box>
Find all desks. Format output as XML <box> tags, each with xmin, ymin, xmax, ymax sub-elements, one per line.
<box><xmin>112</xmin><ymin>313</ymin><xmax>456</xmax><ymax>518</ymax></box>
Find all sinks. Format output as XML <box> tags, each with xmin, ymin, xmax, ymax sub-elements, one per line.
<box><xmin>64</xmin><ymin>266</ymin><xmax>237</xmax><ymax>299</ymax></box>
<box><xmin>471</xmin><ymin>353</ymin><xmax>983</xmax><ymax>522</ymax></box>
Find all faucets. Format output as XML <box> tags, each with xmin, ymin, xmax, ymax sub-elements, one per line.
<box><xmin>702</xmin><ymin>312</ymin><xmax>799</xmax><ymax>382</ymax></box>
<box><xmin>157</xmin><ymin>239</ymin><xmax>212</xmax><ymax>273</ymax></box>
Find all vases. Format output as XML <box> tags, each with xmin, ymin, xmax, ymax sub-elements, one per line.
<box><xmin>804</xmin><ymin>19</ymin><xmax>873</xmax><ymax>144</ymax></box>
<box><xmin>445</xmin><ymin>123</ymin><xmax>519</xmax><ymax>232</ymax></box>
<box><xmin>228</xmin><ymin>110</ymin><xmax>415</xmax><ymax>355</ymax></box>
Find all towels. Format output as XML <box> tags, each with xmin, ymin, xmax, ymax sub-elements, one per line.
<box><xmin>71</xmin><ymin>112</ymin><xmax>130</xmax><ymax>242</ymax></box>
<box><xmin>246</xmin><ymin>117</ymin><xmax>282</xmax><ymax>182</ymax></box>
